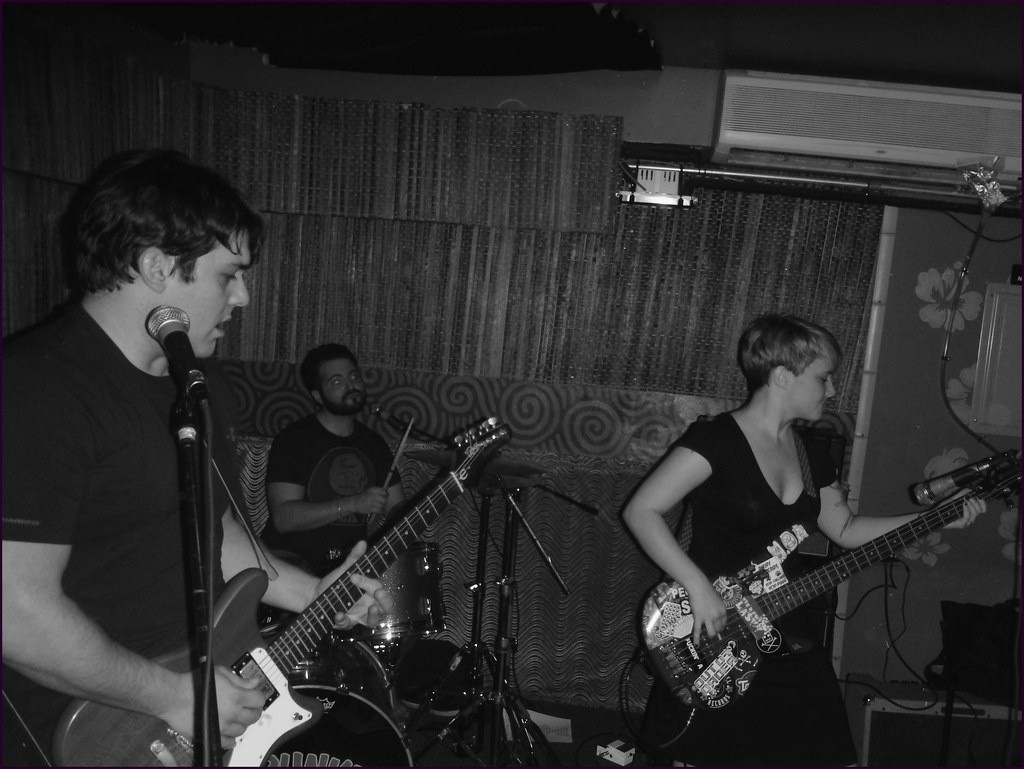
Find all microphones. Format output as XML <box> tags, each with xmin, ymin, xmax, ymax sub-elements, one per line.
<box><xmin>371</xmin><ymin>404</ymin><xmax>411</xmax><ymax>436</ymax></box>
<box><xmin>145</xmin><ymin>304</ymin><xmax>208</xmax><ymax>404</ymax></box>
<box><xmin>914</xmin><ymin>449</ymin><xmax>1017</xmax><ymax>506</ymax></box>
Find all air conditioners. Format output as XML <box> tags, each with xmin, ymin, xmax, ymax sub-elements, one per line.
<box><xmin>711</xmin><ymin>69</ymin><xmax>1024</xmax><ymax>194</ymax></box>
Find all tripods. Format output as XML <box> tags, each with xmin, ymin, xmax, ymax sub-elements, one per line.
<box><xmin>407</xmin><ymin>427</ymin><xmax>566</xmax><ymax>766</ymax></box>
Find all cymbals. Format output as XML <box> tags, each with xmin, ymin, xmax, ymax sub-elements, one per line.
<box><xmin>403</xmin><ymin>444</ymin><xmax>544</xmax><ymax>494</ymax></box>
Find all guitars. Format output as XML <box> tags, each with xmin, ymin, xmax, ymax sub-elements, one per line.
<box><xmin>52</xmin><ymin>416</ymin><xmax>513</xmax><ymax>768</ymax></box>
<box><xmin>639</xmin><ymin>472</ymin><xmax>1023</xmax><ymax>718</ymax></box>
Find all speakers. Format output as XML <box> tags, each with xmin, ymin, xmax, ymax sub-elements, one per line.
<box><xmin>843</xmin><ymin>673</ymin><xmax>1022</xmax><ymax>766</ymax></box>
<box><xmin>768</xmin><ymin>570</ymin><xmax>837</xmax><ymax>666</ymax></box>
<box><xmin>696</xmin><ymin>413</ymin><xmax>847</xmax><ymax>572</ymax></box>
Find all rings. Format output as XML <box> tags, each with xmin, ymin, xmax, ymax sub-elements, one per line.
<box><xmin>969</xmin><ymin>519</ymin><xmax>976</xmax><ymax>524</ymax></box>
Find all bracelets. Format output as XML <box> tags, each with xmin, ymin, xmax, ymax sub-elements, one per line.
<box><xmin>335</xmin><ymin>497</ymin><xmax>344</xmax><ymax>519</ymax></box>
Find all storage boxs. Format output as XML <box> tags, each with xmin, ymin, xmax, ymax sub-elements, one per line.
<box><xmin>844</xmin><ymin>678</ymin><xmax>1022</xmax><ymax>769</ymax></box>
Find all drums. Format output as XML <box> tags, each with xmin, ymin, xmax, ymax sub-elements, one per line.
<box><xmin>262</xmin><ymin>627</ymin><xmax>414</xmax><ymax>768</ymax></box>
<box><xmin>329</xmin><ymin>540</ymin><xmax>445</xmax><ymax>638</ymax></box>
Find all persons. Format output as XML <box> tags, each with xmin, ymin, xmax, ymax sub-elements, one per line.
<box><xmin>2</xmin><ymin>152</ymin><xmax>393</xmax><ymax>767</ymax></box>
<box><xmin>261</xmin><ymin>344</ymin><xmax>409</xmax><ymax>581</ymax></box>
<box><xmin>623</xmin><ymin>316</ymin><xmax>987</xmax><ymax>767</ymax></box>
